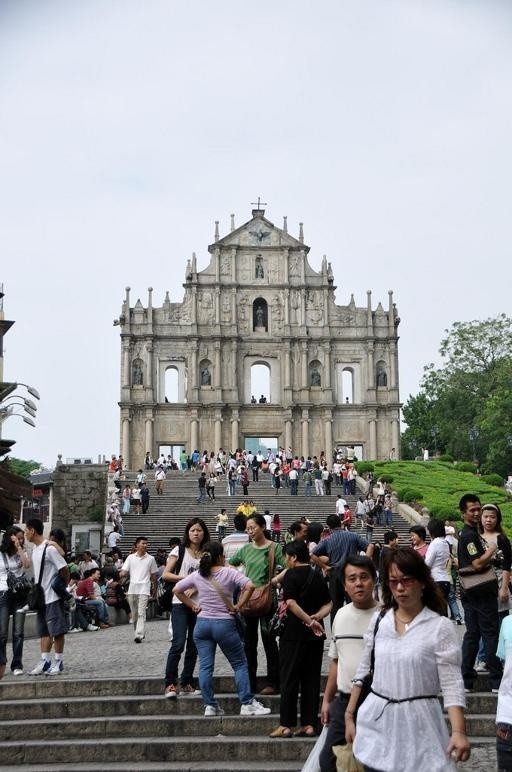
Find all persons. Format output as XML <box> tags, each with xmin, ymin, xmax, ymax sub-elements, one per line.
<box><xmin>377</xmin><ymin>366</ymin><xmax>386</xmax><ymax>386</ymax></box>
<box><xmin>258</xmin><ymin>264</ymin><xmax>263</xmax><ymax>278</ymax></box>
<box><xmin>201</xmin><ymin>366</ymin><xmax>211</xmax><ymax>385</ymax></box>
<box><xmin>136</xmin><ymin>367</ymin><xmax>142</xmax><ymax>385</ymax></box>
<box><xmin>311</xmin><ymin>368</ymin><xmax>320</xmax><ymax>386</ymax></box>
<box><xmin>255</xmin><ymin>306</ymin><xmax>263</xmax><ymax>327</ymax></box>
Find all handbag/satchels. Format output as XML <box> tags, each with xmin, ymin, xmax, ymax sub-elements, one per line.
<box><xmin>353</xmin><ymin>676</ymin><xmax>373</xmax><ymax>723</ymax></box>
<box><xmin>28</xmin><ymin>583</ymin><xmax>44</xmax><ymax>611</ymax></box>
<box><xmin>7</xmin><ymin>572</ymin><xmax>33</xmax><ymax>605</ymax></box>
<box><xmin>266</xmin><ymin>601</ymin><xmax>288</xmax><ymax>632</ymax></box>
<box><xmin>156</xmin><ymin>575</ymin><xmax>175</xmax><ymax>611</ymax></box>
<box><xmin>234</xmin><ymin>612</ymin><xmax>249</xmax><ymax>637</ymax></box>
<box><xmin>239</xmin><ymin>582</ymin><xmax>272</xmax><ymax>610</ymax></box>
<box><xmin>457</xmin><ymin>563</ymin><xmax>496</xmax><ymax>589</ymax></box>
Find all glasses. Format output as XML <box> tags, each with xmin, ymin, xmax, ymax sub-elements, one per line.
<box><xmin>388</xmin><ymin>577</ymin><xmax>413</xmax><ymax>590</ymax></box>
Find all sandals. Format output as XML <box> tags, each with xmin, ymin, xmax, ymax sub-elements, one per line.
<box><xmin>294</xmin><ymin>726</ymin><xmax>316</xmax><ymax>737</ymax></box>
<box><xmin>271</xmin><ymin>727</ymin><xmax>292</xmax><ymax>738</ymax></box>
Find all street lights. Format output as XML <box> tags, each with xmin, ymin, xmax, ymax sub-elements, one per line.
<box><xmin>430</xmin><ymin>424</ymin><xmax>440</xmax><ymax>455</ymax></box>
<box><xmin>408</xmin><ymin>434</ymin><xmax>418</xmax><ymax>459</ymax></box>
<box><xmin>468</xmin><ymin>425</ymin><xmax>480</xmax><ymax>461</ymax></box>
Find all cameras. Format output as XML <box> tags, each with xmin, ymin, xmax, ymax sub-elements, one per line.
<box><xmin>495</xmin><ymin>550</ymin><xmax>504</xmax><ymax>561</ymax></box>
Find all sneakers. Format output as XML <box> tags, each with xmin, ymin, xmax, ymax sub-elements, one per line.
<box><xmin>476</xmin><ymin>662</ymin><xmax>490</xmax><ymax>672</ymax></box>
<box><xmin>205</xmin><ymin>706</ymin><xmax>225</xmax><ymax>716</ymax></box>
<box><xmin>69</xmin><ymin>600</ymin><xmax>76</xmax><ymax>612</ymax></box>
<box><xmin>134</xmin><ymin>638</ymin><xmax>142</xmax><ymax>643</ymax></box>
<box><xmin>69</xmin><ymin>621</ymin><xmax>114</xmax><ymax>635</ymax></box>
<box><xmin>13</xmin><ymin>669</ymin><xmax>23</xmax><ymax>676</ymax></box>
<box><xmin>260</xmin><ymin>685</ymin><xmax>279</xmax><ymax>695</ymax></box>
<box><xmin>241</xmin><ymin>699</ymin><xmax>271</xmax><ymax>716</ymax></box>
<box><xmin>28</xmin><ymin>658</ymin><xmax>51</xmax><ymax>676</ymax></box>
<box><xmin>165</xmin><ymin>685</ymin><xmax>177</xmax><ymax>698</ymax></box>
<box><xmin>180</xmin><ymin>685</ymin><xmax>202</xmax><ymax>696</ymax></box>
<box><xmin>44</xmin><ymin>659</ymin><xmax>63</xmax><ymax>676</ymax></box>
